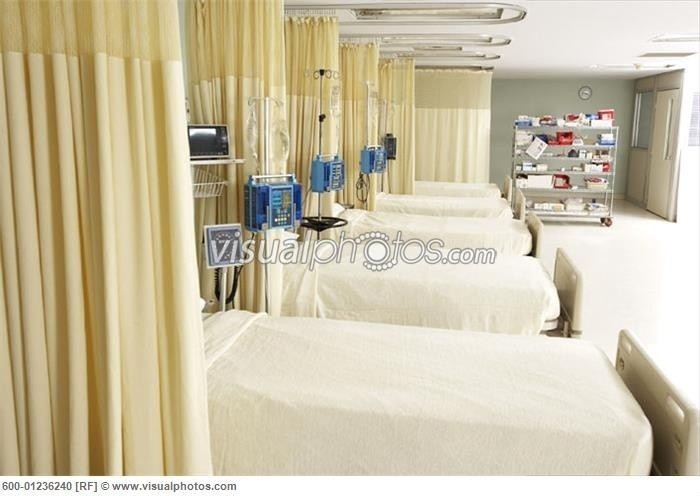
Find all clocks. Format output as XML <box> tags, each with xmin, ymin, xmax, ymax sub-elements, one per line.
<box><xmin>578</xmin><ymin>86</ymin><xmax>594</xmax><ymax>101</ymax></box>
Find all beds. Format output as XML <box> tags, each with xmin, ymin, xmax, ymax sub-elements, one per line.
<box><xmin>197</xmin><ymin>180</ymin><xmax>699</xmax><ymax>475</ymax></box>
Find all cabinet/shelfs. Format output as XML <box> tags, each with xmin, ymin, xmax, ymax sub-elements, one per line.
<box><xmin>510</xmin><ymin>121</ymin><xmax>620</xmax><ymax>227</ymax></box>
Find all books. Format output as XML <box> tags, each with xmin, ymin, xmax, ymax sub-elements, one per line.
<box><xmin>526</xmin><ymin>137</ymin><xmax>547</xmax><ymax>160</ymax></box>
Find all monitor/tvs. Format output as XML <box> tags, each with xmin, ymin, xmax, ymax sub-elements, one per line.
<box><xmin>188</xmin><ymin>123</ymin><xmax>231</xmax><ymax>159</ymax></box>
